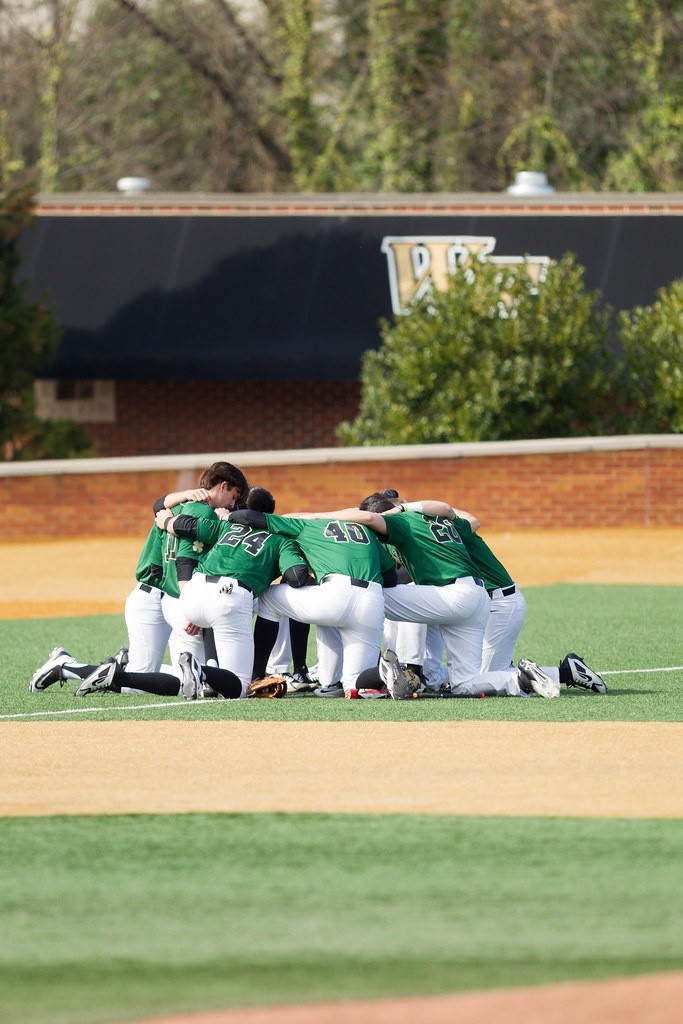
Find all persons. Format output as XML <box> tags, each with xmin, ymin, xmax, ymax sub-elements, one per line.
<box><xmin>275</xmin><ymin>489</ymin><xmax>562</xmax><ymax>701</ymax></box>
<box><xmin>156</xmin><ymin>486</ymin><xmax>462</xmax><ymax>691</ymax></box>
<box><xmin>378</xmin><ymin>489</ymin><xmax>608</xmax><ymax>698</ymax></box>
<box><xmin>29</xmin><ymin>463</ymin><xmax>250</xmax><ymax>699</ymax></box>
<box><xmin>74</xmin><ymin>461</ymin><xmax>249</xmax><ymax>706</ymax></box>
<box><xmin>152</xmin><ymin>485</ymin><xmax>310</xmax><ymax>703</ymax></box>
<box><xmin>212</xmin><ymin>507</ymin><xmax>409</xmax><ymax>702</ymax></box>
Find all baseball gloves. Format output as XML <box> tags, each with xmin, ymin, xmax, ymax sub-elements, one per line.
<box><xmin>246</xmin><ymin>675</ymin><xmax>287</xmax><ymax>700</ymax></box>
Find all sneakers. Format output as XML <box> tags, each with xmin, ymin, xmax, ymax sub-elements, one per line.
<box><xmin>29</xmin><ymin>646</ymin><xmax>608</xmax><ymax>702</ymax></box>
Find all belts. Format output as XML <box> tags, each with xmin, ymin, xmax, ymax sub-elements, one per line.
<box><xmin>321</xmin><ymin>575</ymin><xmax>369</xmax><ymax>589</ymax></box>
<box><xmin>489</xmin><ymin>585</ymin><xmax>516</xmax><ymax>599</ymax></box>
<box><xmin>205</xmin><ymin>576</ymin><xmax>252</xmax><ymax>593</ymax></box>
<box><xmin>448</xmin><ymin>577</ymin><xmax>484</xmax><ymax>588</ymax></box>
<box><xmin>140</xmin><ymin>584</ymin><xmax>165</xmax><ymax>600</ymax></box>
